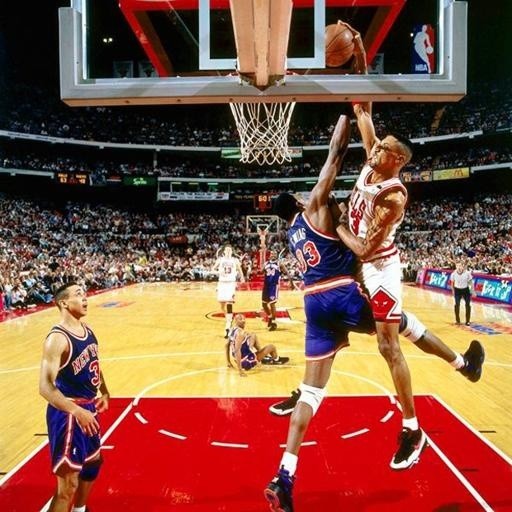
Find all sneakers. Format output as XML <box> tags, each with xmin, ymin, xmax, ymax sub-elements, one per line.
<box><xmin>390</xmin><ymin>427</ymin><xmax>425</xmax><ymax>469</ymax></box>
<box><xmin>264</xmin><ymin>470</ymin><xmax>295</xmax><ymax>512</ymax></box>
<box><xmin>456</xmin><ymin>341</ymin><xmax>484</xmax><ymax>382</ymax></box>
<box><xmin>269</xmin><ymin>389</ymin><xmax>302</xmax><ymax>416</ymax></box>
<box><xmin>224</xmin><ymin>317</ymin><xmax>289</xmax><ymax>364</ymax></box>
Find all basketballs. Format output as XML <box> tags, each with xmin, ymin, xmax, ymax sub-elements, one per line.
<box><xmin>324</xmin><ymin>24</ymin><xmax>355</xmax><ymax>67</ymax></box>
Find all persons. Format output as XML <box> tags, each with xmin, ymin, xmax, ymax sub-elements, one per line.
<box><xmin>225</xmin><ymin>313</ymin><xmax>290</xmax><ymax>378</ymax></box>
<box><xmin>268</xmin><ymin>19</ymin><xmax>454</xmax><ymax>470</ymax></box>
<box><xmin>258</xmin><ymin>241</ymin><xmax>295</xmax><ymax>330</ymax></box>
<box><xmin>38</xmin><ymin>281</ymin><xmax>110</xmax><ymax>512</ymax></box>
<box><xmin>211</xmin><ymin>245</ymin><xmax>244</xmax><ymax>338</ymax></box>
<box><xmin>263</xmin><ymin>103</ymin><xmax>486</xmax><ymax>512</ymax></box>
<box><xmin>449</xmin><ymin>260</ymin><xmax>474</xmax><ymax>326</ymax></box>
<box><xmin>1</xmin><ymin>93</ymin><xmax>511</xmax><ymax>314</ymax></box>
